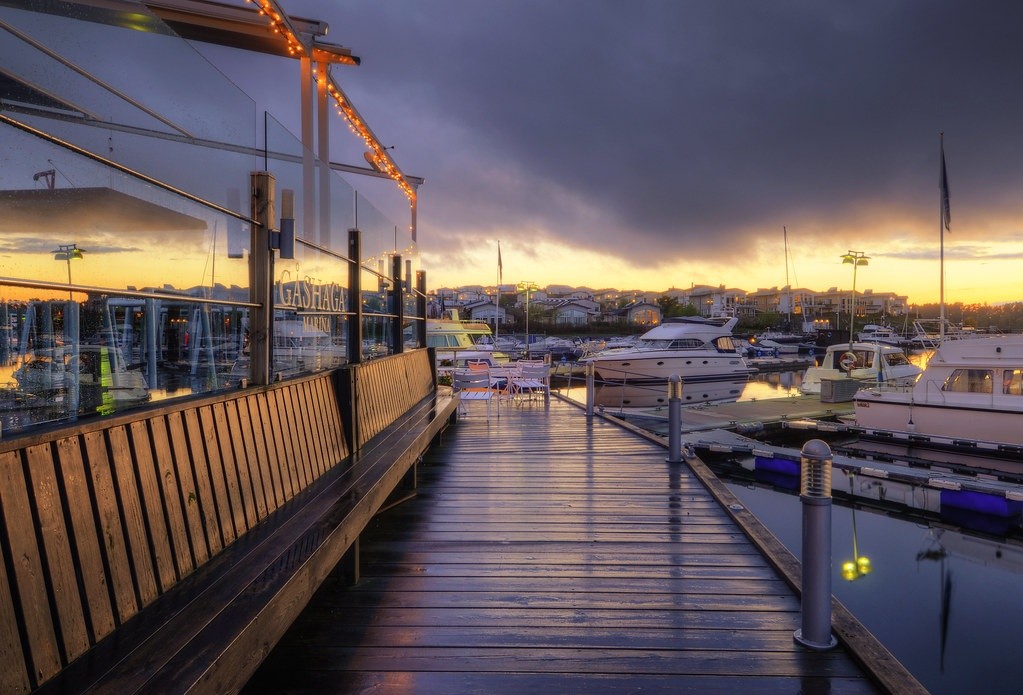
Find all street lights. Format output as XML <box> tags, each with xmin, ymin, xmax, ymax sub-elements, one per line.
<box><xmin>517</xmin><ymin>280</ymin><xmax>538</xmax><ymax>359</ymax></box>
<box><xmin>840</xmin><ymin>250</ymin><xmax>872</xmax><ymax>376</ymax></box>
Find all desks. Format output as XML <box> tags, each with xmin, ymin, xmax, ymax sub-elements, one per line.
<box><xmin>480</xmin><ymin>368</ymin><xmax>530</xmax><ymax>405</ymax></box>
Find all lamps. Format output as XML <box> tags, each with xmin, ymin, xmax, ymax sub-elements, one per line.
<box><xmin>269</xmin><ymin>217</ymin><xmax>295</xmax><ymax>260</ymax></box>
<box><xmin>402</xmin><ymin>274</ymin><xmax>411</xmax><ymax>293</ymax></box>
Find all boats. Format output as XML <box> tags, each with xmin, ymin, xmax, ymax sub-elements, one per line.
<box><xmin>857</xmin><ymin>313</ymin><xmax>907</xmax><ymax>346</ymax></box>
<box><xmin>594</xmin><ymin>380</ymin><xmax>750</xmax><ymax>410</ymax></box>
<box><xmin>493</xmin><ymin>336</ymin><xmax>636</xmax><ymax>360</ymax></box>
<box><xmin>227</xmin><ymin>316</ymin><xmax>389</xmax><ymax>383</ymax></box>
<box><xmin>427</xmin><ymin>307</ymin><xmax>510</xmax><ymax>376</ymax></box>
<box><xmin>743</xmin><ymin>336</ymin><xmax>799</xmax><ymax>356</ymax></box>
<box><xmin>800</xmin><ymin>341</ymin><xmax>923</xmax><ymax>394</ymax></box>
<box><xmin>579</xmin><ymin>315</ymin><xmax>759</xmax><ymax>383</ymax></box>
<box><xmin>854</xmin><ymin>316</ymin><xmax>1023</xmax><ymax>447</ymax></box>
<box><xmin>910</xmin><ymin>317</ymin><xmax>1005</xmax><ymax>346</ymax></box>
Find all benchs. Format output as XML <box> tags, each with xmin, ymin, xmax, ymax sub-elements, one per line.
<box><xmin>0</xmin><ymin>348</ymin><xmax>467</xmax><ymax>695</ymax></box>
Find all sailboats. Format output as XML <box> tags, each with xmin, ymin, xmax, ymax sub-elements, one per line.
<box><xmin>756</xmin><ymin>226</ymin><xmax>818</xmax><ymax>344</ymax></box>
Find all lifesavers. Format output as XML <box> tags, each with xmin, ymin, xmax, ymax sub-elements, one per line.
<box><xmin>838</xmin><ymin>351</ymin><xmax>857</xmax><ymax>371</ymax></box>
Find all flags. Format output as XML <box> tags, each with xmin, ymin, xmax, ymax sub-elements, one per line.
<box><xmin>940</xmin><ymin>574</ymin><xmax>952</xmax><ymax>667</ymax></box>
<box><xmin>939</xmin><ymin>150</ymin><xmax>951</xmax><ymax>232</ymax></box>
<box><xmin>498</xmin><ymin>244</ymin><xmax>503</xmax><ymax>286</ymax></box>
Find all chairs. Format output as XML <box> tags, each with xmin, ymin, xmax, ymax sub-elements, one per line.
<box><xmin>452</xmin><ymin>358</ymin><xmax>555</xmax><ymax>423</ymax></box>
